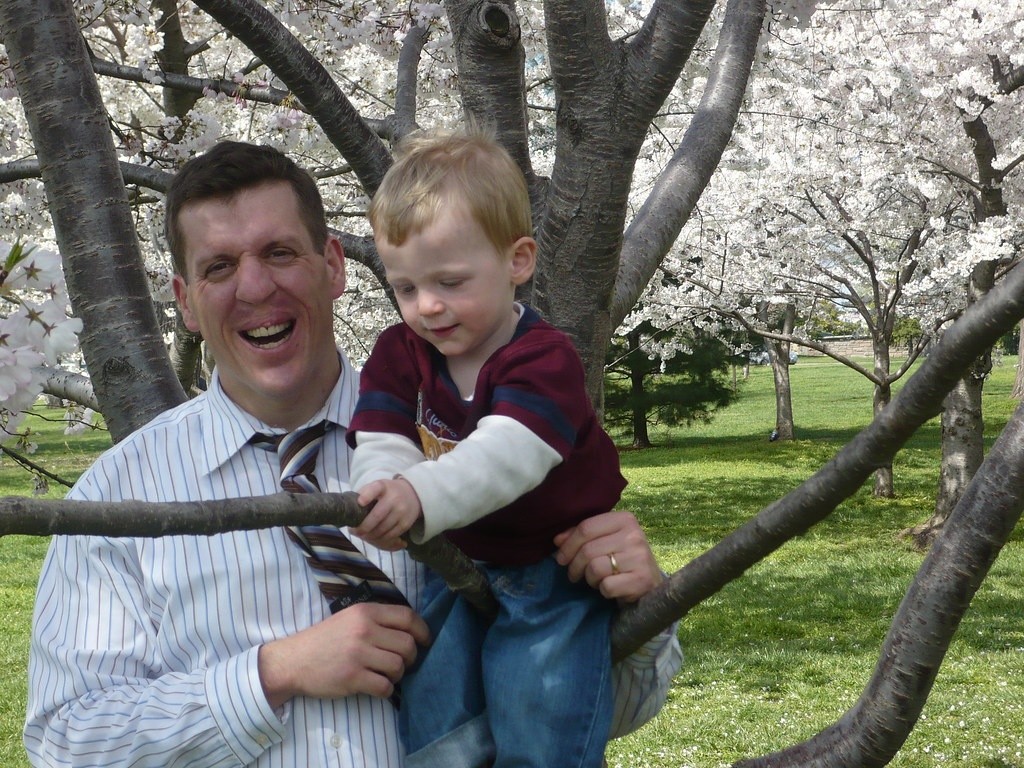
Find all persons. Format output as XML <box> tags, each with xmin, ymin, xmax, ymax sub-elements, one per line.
<box><xmin>346</xmin><ymin>115</ymin><xmax>626</xmax><ymax>768</ymax></box>
<box><xmin>23</xmin><ymin>142</ymin><xmax>683</xmax><ymax>768</ymax></box>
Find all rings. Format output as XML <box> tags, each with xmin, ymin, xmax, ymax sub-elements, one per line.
<box><xmin>604</xmin><ymin>550</ymin><xmax>623</xmax><ymax>577</ymax></box>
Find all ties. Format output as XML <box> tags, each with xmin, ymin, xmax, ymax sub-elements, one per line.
<box><xmin>247</xmin><ymin>419</ymin><xmax>414</xmax><ymax>713</ymax></box>
